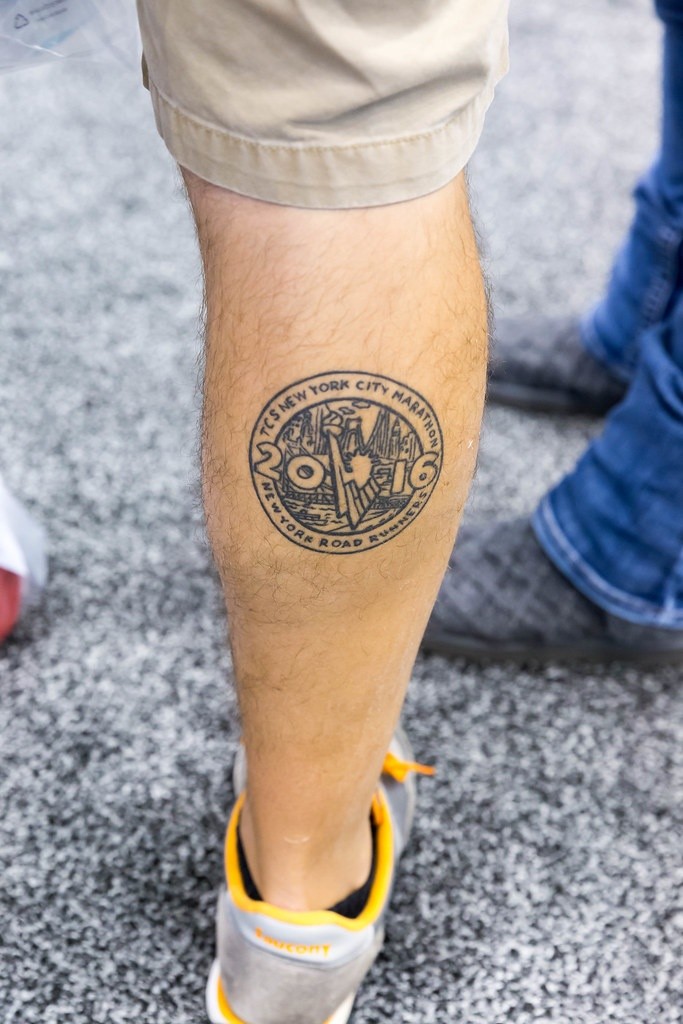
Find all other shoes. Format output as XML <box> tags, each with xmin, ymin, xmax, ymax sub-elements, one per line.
<box><xmin>488</xmin><ymin>299</ymin><xmax>622</xmax><ymax>414</ymax></box>
<box><xmin>419</xmin><ymin>506</ymin><xmax>682</xmax><ymax>662</ymax></box>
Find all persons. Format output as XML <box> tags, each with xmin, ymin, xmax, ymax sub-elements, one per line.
<box><xmin>138</xmin><ymin>0</ymin><xmax>512</xmax><ymax>1024</ymax></box>
<box><xmin>419</xmin><ymin>0</ymin><xmax>682</xmax><ymax>668</ymax></box>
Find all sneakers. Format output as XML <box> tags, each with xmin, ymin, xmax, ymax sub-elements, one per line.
<box><xmin>204</xmin><ymin>715</ymin><xmax>436</xmax><ymax>1023</ymax></box>
